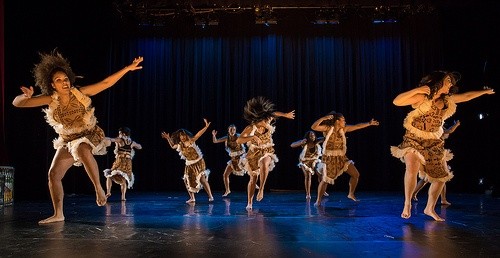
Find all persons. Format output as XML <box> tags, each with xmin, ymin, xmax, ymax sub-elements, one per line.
<box><xmin>161</xmin><ymin>119</ymin><xmax>214</xmax><ymax>203</ymax></box>
<box><xmin>12</xmin><ymin>49</ymin><xmax>143</xmax><ymax>223</ymax></box>
<box><xmin>236</xmin><ymin>98</ymin><xmax>295</xmax><ymax>210</ymax></box>
<box><xmin>212</xmin><ymin>125</ymin><xmax>259</xmax><ymax>196</ymax></box>
<box><xmin>103</xmin><ymin>127</ymin><xmax>142</xmax><ymax>200</ymax></box>
<box><xmin>291</xmin><ymin>131</ymin><xmax>349</xmax><ymax>200</ymax></box>
<box><xmin>390</xmin><ymin>72</ymin><xmax>495</xmax><ymax>221</ymax></box>
<box><xmin>311</xmin><ymin>112</ymin><xmax>379</xmax><ymax>206</ymax></box>
